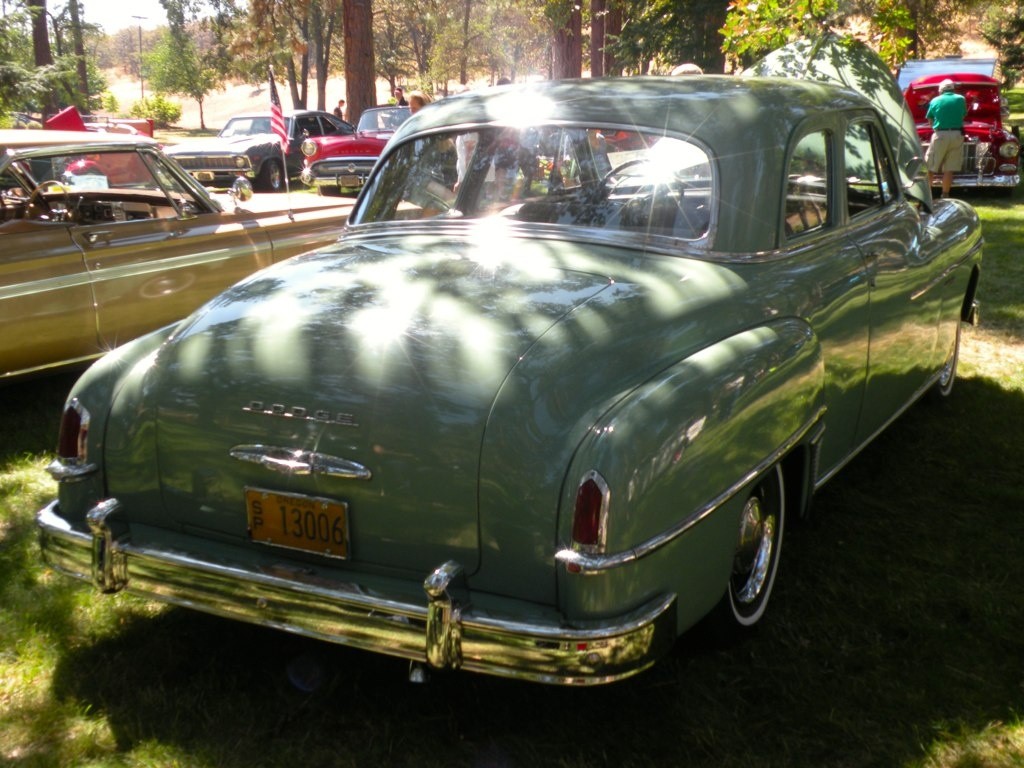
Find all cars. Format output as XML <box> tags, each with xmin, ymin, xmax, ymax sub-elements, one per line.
<box><xmin>8</xmin><ymin>121</ymin><xmax>165</xmax><ymax>187</ymax></box>
<box><xmin>32</xmin><ymin>74</ymin><xmax>987</xmax><ymax>686</ymax></box>
<box><xmin>302</xmin><ymin>104</ymin><xmax>418</xmax><ymax>199</ymax></box>
<box><xmin>899</xmin><ymin>71</ymin><xmax>1021</xmax><ymax>190</ymax></box>
<box><xmin>159</xmin><ymin>111</ymin><xmax>356</xmax><ymax>192</ymax></box>
<box><xmin>0</xmin><ymin>128</ymin><xmax>423</xmax><ymax>388</ymax></box>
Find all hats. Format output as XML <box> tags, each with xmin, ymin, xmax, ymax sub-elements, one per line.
<box><xmin>671</xmin><ymin>63</ymin><xmax>703</xmax><ymax>76</ymax></box>
<box><xmin>939</xmin><ymin>79</ymin><xmax>954</xmax><ymax>92</ymax></box>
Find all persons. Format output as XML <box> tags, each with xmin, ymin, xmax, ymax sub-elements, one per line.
<box><xmin>334</xmin><ymin>100</ymin><xmax>345</xmax><ymax>119</ymax></box>
<box><xmin>924</xmin><ymin>80</ymin><xmax>968</xmax><ymax>197</ymax></box>
<box><xmin>393</xmin><ymin>74</ymin><xmax>611</xmax><ymax>201</ymax></box>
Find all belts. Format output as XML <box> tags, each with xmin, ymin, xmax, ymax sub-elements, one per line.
<box><xmin>938</xmin><ymin>127</ymin><xmax>961</xmax><ymax>131</ymax></box>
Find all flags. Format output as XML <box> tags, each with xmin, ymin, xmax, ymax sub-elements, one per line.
<box><xmin>268</xmin><ymin>69</ymin><xmax>291</xmax><ymax>154</ymax></box>
